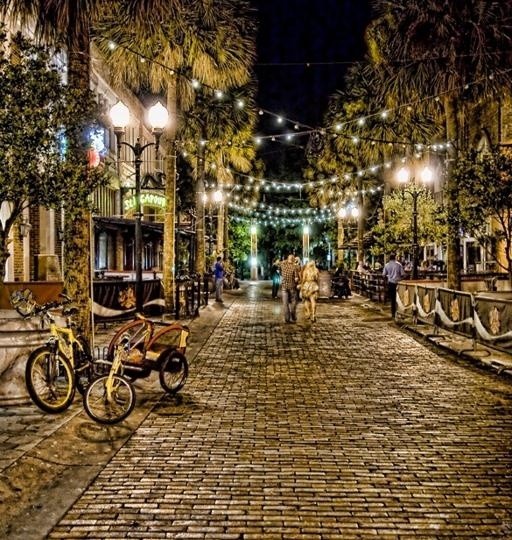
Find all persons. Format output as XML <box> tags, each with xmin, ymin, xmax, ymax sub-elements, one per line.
<box><xmin>270</xmin><ymin>250</ymin><xmax>445</xmax><ymax>324</ymax></box>
<box><xmin>213</xmin><ymin>255</ymin><xmax>228</xmax><ymax>304</ymax></box>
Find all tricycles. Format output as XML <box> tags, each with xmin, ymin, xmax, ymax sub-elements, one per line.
<box><xmin>83</xmin><ymin>310</ymin><xmax>191</xmax><ymax>423</ymax></box>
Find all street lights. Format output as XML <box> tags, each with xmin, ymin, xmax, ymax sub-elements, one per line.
<box><xmin>201</xmin><ymin>190</ymin><xmax>226</xmax><ymax>266</ymax></box>
<box><xmin>338</xmin><ymin>207</ymin><xmax>360</xmax><ymax>269</ymax></box>
<box><xmin>395</xmin><ymin>164</ymin><xmax>433</xmax><ymax>281</ymax></box>
<box><xmin>109</xmin><ymin>99</ymin><xmax>169</xmax><ymax>319</ymax></box>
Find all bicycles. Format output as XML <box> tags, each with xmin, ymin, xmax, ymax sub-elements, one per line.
<box><xmin>11</xmin><ymin>290</ymin><xmax>96</xmax><ymax>414</ymax></box>
